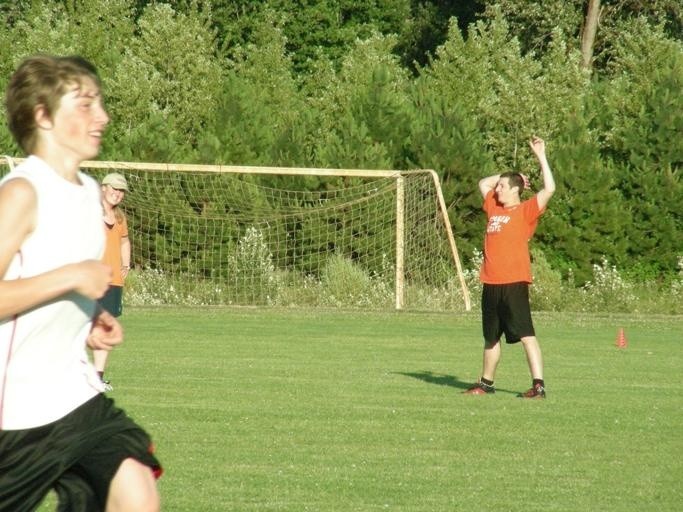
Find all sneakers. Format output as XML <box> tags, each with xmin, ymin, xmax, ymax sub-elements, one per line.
<box><xmin>102</xmin><ymin>380</ymin><xmax>113</xmax><ymax>393</ymax></box>
<box><xmin>511</xmin><ymin>387</ymin><xmax>545</xmax><ymax>402</ymax></box>
<box><xmin>456</xmin><ymin>386</ymin><xmax>495</xmax><ymax>395</ymax></box>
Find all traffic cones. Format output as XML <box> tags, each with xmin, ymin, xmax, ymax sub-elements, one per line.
<box><xmin>617</xmin><ymin>329</ymin><xmax>626</xmax><ymax>348</ymax></box>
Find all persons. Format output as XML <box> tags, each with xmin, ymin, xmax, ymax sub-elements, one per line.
<box><xmin>461</xmin><ymin>133</ymin><xmax>557</xmax><ymax>397</ymax></box>
<box><xmin>91</xmin><ymin>172</ymin><xmax>132</xmax><ymax>392</ymax></box>
<box><xmin>0</xmin><ymin>53</ymin><xmax>164</xmax><ymax>512</ymax></box>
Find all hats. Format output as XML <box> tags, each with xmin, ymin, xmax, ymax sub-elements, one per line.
<box><xmin>102</xmin><ymin>173</ymin><xmax>130</xmax><ymax>193</ymax></box>
